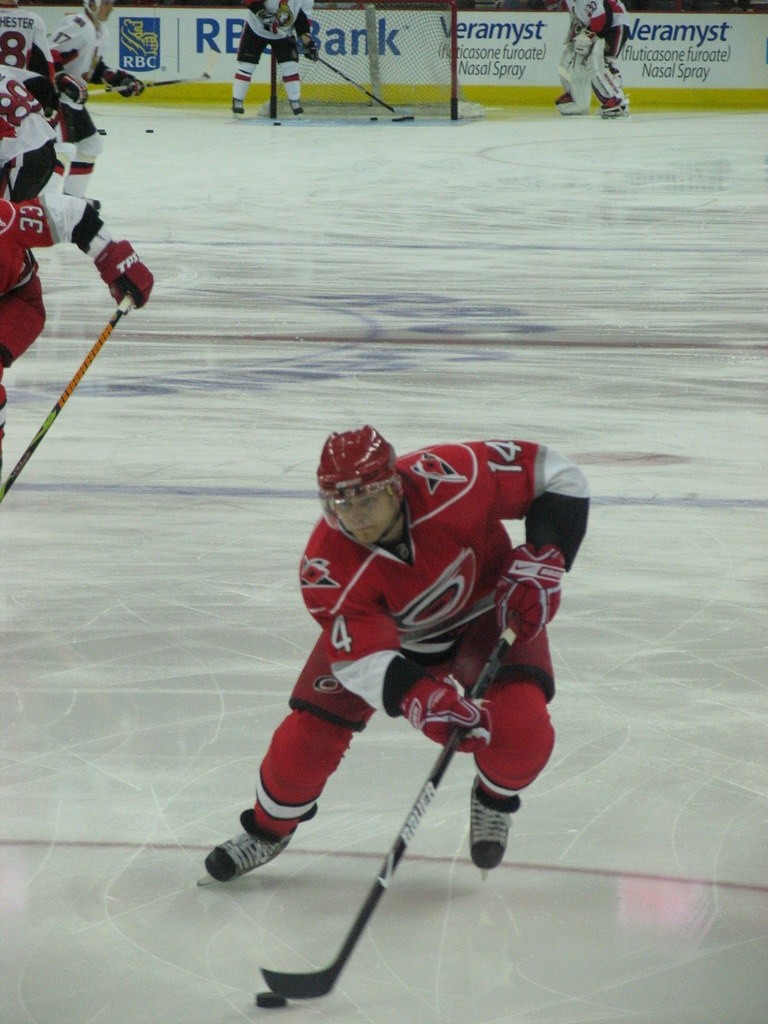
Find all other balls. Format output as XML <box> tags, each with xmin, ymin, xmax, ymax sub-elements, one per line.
<box><xmin>393</xmin><ymin>117</ymin><xmax>414</xmax><ymax>122</ymax></box>
<box><xmin>255</xmin><ymin>992</ymin><xmax>287</xmax><ymax>1008</ymax></box>
<box><xmin>98</xmin><ymin>129</ymin><xmax>106</xmax><ymax>135</ymax></box>
<box><xmin>370</xmin><ymin>117</ymin><xmax>377</xmax><ymax>120</ymax></box>
<box><xmin>146</xmin><ymin>130</ymin><xmax>154</xmax><ymax>133</ymax></box>
<box><xmin>273</xmin><ymin>122</ymin><xmax>281</xmax><ymax>126</ymax></box>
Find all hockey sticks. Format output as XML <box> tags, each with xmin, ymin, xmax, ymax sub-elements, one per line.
<box><xmin>276</xmin><ymin>27</ymin><xmax>413</xmax><ymax>115</ymax></box>
<box><xmin>558</xmin><ymin>52</ymin><xmax>579</xmax><ymax>84</ymax></box>
<box><xmin>0</xmin><ymin>293</ymin><xmax>134</xmax><ymax>503</ymax></box>
<box><xmin>87</xmin><ymin>49</ymin><xmax>219</xmax><ymax>96</ymax></box>
<box><xmin>261</xmin><ymin>608</ymin><xmax>523</xmax><ymax>1000</ymax></box>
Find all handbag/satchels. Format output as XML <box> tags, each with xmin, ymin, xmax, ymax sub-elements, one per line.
<box><xmin>496</xmin><ymin>545</ymin><xmax>567</xmax><ymax>645</ymax></box>
<box><xmin>397</xmin><ymin>677</ymin><xmax>493</xmax><ymax>751</ymax></box>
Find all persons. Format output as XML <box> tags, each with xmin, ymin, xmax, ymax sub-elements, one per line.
<box><xmin>555</xmin><ymin>0</ymin><xmax>632</xmax><ymax>121</ymax></box>
<box><xmin>231</xmin><ymin>0</ymin><xmax>319</xmax><ymax>120</ymax></box>
<box><xmin>0</xmin><ymin>0</ymin><xmax>154</xmax><ymax>485</ymax></box>
<box><xmin>154</xmin><ymin>0</ymin><xmax>768</xmax><ymax>14</ymax></box>
<box><xmin>197</xmin><ymin>425</ymin><xmax>590</xmax><ymax>888</ymax></box>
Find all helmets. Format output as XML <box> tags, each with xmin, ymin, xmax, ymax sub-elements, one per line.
<box><xmin>317</xmin><ymin>425</ymin><xmax>395</xmax><ymax>500</ymax></box>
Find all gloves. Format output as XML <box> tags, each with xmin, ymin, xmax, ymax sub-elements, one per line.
<box><xmin>95</xmin><ymin>237</ymin><xmax>153</xmax><ymax>309</ymax></box>
<box><xmin>302</xmin><ymin>33</ymin><xmax>317</xmax><ymax>62</ymax></box>
<box><xmin>107</xmin><ymin>70</ymin><xmax>144</xmax><ymax>97</ymax></box>
<box><xmin>260</xmin><ymin>11</ymin><xmax>280</xmax><ymax>36</ymax></box>
<box><xmin>57</xmin><ymin>74</ymin><xmax>88</xmax><ymax>105</ymax></box>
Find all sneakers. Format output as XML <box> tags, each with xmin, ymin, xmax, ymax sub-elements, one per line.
<box><xmin>290</xmin><ymin>100</ymin><xmax>304</xmax><ymax>119</ymax></box>
<box><xmin>232</xmin><ymin>97</ymin><xmax>244</xmax><ymax>119</ymax></box>
<box><xmin>471</xmin><ymin>773</ymin><xmax>519</xmax><ymax>881</ymax></box>
<box><xmin>196</xmin><ymin>802</ymin><xmax>319</xmax><ymax>886</ymax></box>
